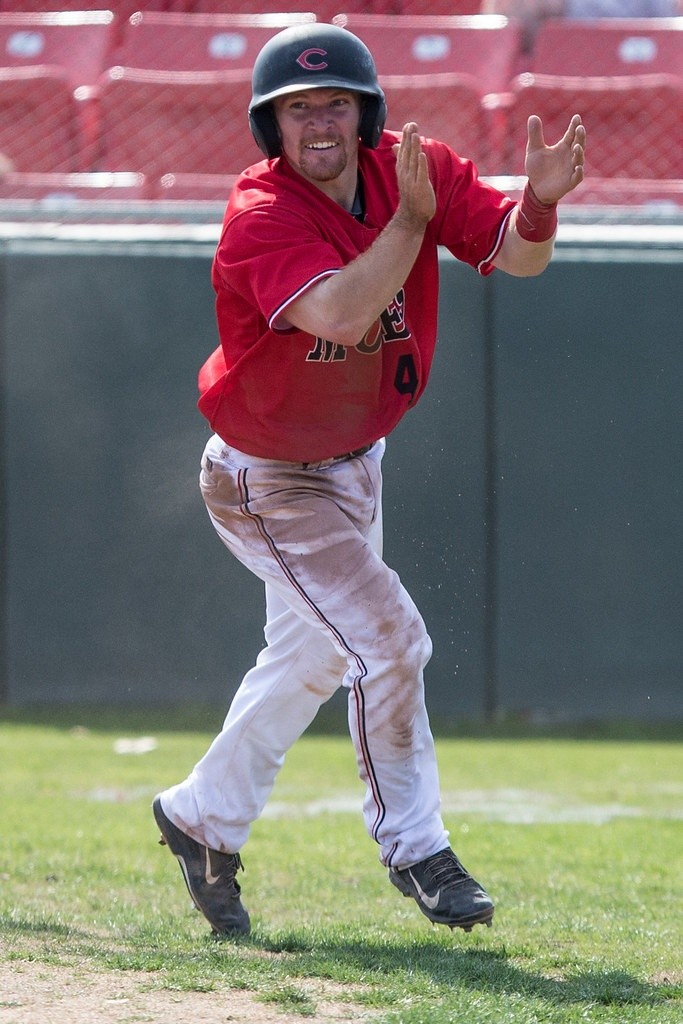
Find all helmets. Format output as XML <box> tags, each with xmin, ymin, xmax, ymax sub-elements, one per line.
<box><xmin>248</xmin><ymin>23</ymin><xmax>387</xmax><ymax>161</ymax></box>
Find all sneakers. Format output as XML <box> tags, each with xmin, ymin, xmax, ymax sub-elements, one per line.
<box><xmin>153</xmin><ymin>792</ymin><xmax>251</xmax><ymax>937</ymax></box>
<box><xmin>389</xmin><ymin>846</ymin><xmax>495</xmax><ymax>932</ymax></box>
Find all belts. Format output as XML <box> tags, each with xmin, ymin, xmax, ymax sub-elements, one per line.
<box><xmin>301</xmin><ymin>441</ymin><xmax>377</xmax><ymax>471</ymax></box>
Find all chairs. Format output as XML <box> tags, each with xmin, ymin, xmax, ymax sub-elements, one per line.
<box><xmin>0</xmin><ymin>1</ymin><xmax>683</xmax><ymax>206</ymax></box>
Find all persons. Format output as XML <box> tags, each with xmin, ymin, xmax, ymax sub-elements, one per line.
<box><xmin>152</xmin><ymin>23</ymin><xmax>585</xmax><ymax>939</ymax></box>
